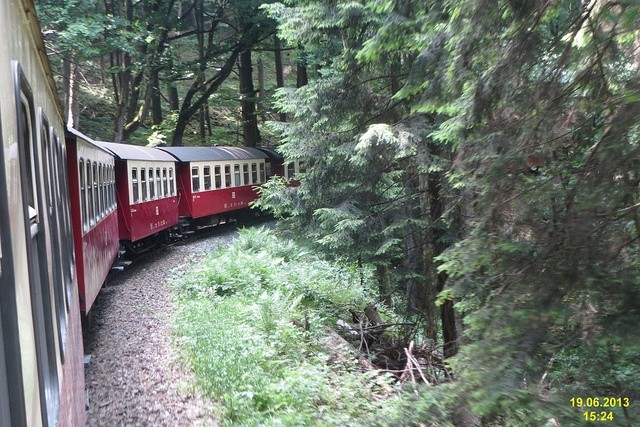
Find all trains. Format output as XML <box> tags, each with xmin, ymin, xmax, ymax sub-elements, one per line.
<box><xmin>1</xmin><ymin>0</ymin><xmax>304</xmax><ymax>427</ymax></box>
